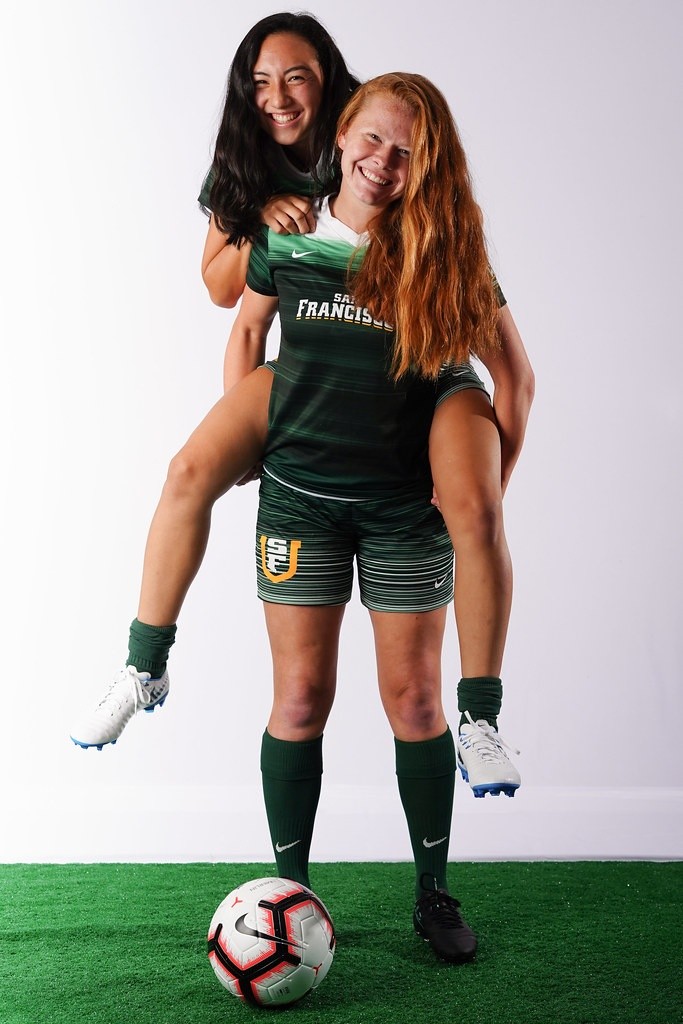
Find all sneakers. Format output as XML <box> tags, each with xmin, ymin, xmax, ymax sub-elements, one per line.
<box><xmin>456</xmin><ymin>719</ymin><xmax>521</xmax><ymax>797</ymax></box>
<box><xmin>413</xmin><ymin>889</ymin><xmax>477</xmax><ymax>964</ymax></box>
<box><xmin>71</xmin><ymin>663</ymin><xmax>170</xmax><ymax>751</ymax></box>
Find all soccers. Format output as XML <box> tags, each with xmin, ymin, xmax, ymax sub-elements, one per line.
<box><xmin>207</xmin><ymin>877</ymin><xmax>337</xmax><ymax>1008</ymax></box>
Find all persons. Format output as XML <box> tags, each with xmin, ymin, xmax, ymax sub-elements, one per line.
<box><xmin>219</xmin><ymin>73</ymin><xmax>536</xmax><ymax>968</ymax></box>
<box><xmin>67</xmin><ymin>12</ymin><xmax>522</xmax><ymax>799</ymax></box>
